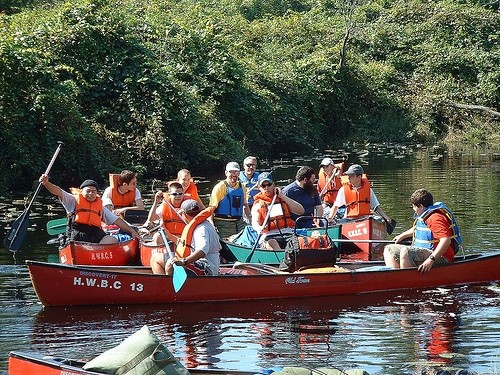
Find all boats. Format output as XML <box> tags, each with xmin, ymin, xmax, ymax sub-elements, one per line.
<box><xmin>8</xmin><ymin>349</ymin><xmax>276</xmax><ymax>375</ymax></box>
<box><xmin>26</xmin><ymin>249</ymin><xmax>500</xmax><ymax>311</ymax></box>
<box><xmin>139</xmin><ymin>232</ymin><xmax>180</xmax><ymax>267</ymax></box>
<box><xmin>221</xmin><ymin>233</ymin><xmax>338</xmax><ymax>265</ymax></box>
<box><xmin>292</xmin><ymin>221</ymin><xmax>343</xmax><ymax>261</ymax></box>
<box><xmin>57</xmin><ymin>234</ymin><xmax>141</xmax><ymax>266</ymax></box>
<box><xmin>319</xmin><ymin>212</ymin><xmax>387</xmax><ymax>256</ymax></box>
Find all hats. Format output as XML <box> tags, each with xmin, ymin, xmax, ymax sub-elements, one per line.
<box><xmin>258</xmin><ymin>171</ymin><xmax>272</xmax><ymax>186</ymax></box>
<box><xmin>79</xmin><ymin>179</ymin><xmax>100</xmax><ymax>192</ymax></box>
<box><xmin>177</xmin><ymin>198</ymin><xmax>200</xmax><ymax>214</ymax></box>
<box><xmin>226</xmin><ymin>161</ymin><xmax>240</xmax><ymax>172</ymax></box>
<box><xmin>319</xmin><ymin>158</ymin><xmax>334</xmax><ymax>167</ymax></box>
<box><xmin>343</xmin><ymin>164</ymin><xmax>363</xmax><ymax>174</ymax></box>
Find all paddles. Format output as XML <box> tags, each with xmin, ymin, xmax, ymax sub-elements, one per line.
<box><xmin>3</xmin><ymin>141</ymin><xmax>65</xmax><ymax>252</ymax></box>
<box><xmin>245</xmin><ymin>191</ymin><xmax>279</xmax><ymax>263</ymax></box>
<box><xmin>331</xmin><ymin>237</ymin><xmax>412</xmax><ymax>246</ymax></box>
<box><xmin>46</xmin><ymin>216</ymin><xmax>69</xmax><ymax>236</ymax></box>
<box><xmin>159</xmin><ymin>227</ymin><xmax>188</xmax><ymax>293</ymax></box>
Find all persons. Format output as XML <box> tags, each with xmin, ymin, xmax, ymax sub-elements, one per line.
<box><xmin>317</xmin><ymin>158</ymin><xmax>342</xmax><ymax>222</ymax></box>
<box><xmin>251</xmin><ymin>171</ymin><xmax>306</xmax><ymax>251</ymax></box>
<box><xmin>329</xmin><ymin>165</ymin><xmax>391</xmax><ymax>225</ymax></box>
<box><xmin>101</xmin><ymin>169</ymin><xmax>145</xmax><ymax>224</ymax></box>
<box><xmin>281</xmin><ymin>166</ymin><xmax>323</xmax><ymax>229</ymax></box>
<box><xmin>383</xmin><ymin>189</ymin><xmax>454</xmax><ymax>273</ymax></box>
<box><xmin>39</xmin><ymin>173</ymin><xmax>140</xmax><ymax>244</ymax></box>
<box><xmin>167</xmin><ymin>169</ymin><xmax>216</xmax><ymax>230</ymax></box>
<box><xmin>208</xmin><ymin>161</ymin><xmax>252</xmax><ymax>231</ymax></box>
<box><xmin>238</xmin><ymin>156</ymin><xmax>261</xmax><ymax>222</ymax></box>
<box><xmin>148</xmin><ymin>183</ymin><xmax>188</xmax><ymax>245</ymax></box>
<box><xmin>149</xmin><ymin>198</ymin><xmax>220</xmax><ymax>276</ymax></box>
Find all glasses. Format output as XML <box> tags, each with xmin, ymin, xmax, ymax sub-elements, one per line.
<box><xmin>168</xmin><ymin>192</ymin><xmax>185</xmax><ymax>196</ymax></box>
<box><xmin>244</xmin><ymin>163</ymin><xmax>256</xmax><ymax>168</ymax></box>
<box><xmin>262</xmin><ymin>182</ymin><xmax>272</xmax><ymax>187</ymax></box>
